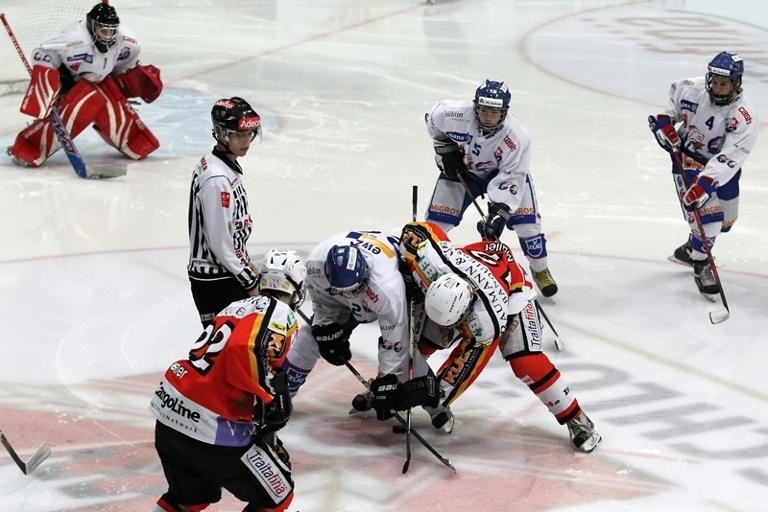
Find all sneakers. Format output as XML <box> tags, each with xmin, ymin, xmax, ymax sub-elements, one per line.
<box><xmin>422</xmin><ymin>389</ymin><xmax>451</xmax><ymax>429</ymax></box>
<box><xmin>571</xmin><ymin>411</ymin><xmax>593</xmax><ymax>448</ymax></box>
<box><xmin>675</xmin><ymin>243</ymin><xmax>720</xmax><ymax>294</ymax></box>
<box><xmin>530</xmin><ymin>266</ymin><xmax>557</xmax><ymax>297</ymax></box>
<box><xmin>352</xmin><ymin>391</ymin><xmax>374</xmax><ymax>411</ymax></box>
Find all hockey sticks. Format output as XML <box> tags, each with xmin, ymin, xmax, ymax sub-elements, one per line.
<box><xmin>673</xmin><ymin>145</ymin><xmax>730</xmax><ymax>325</ymax></box>
<box><xmin>402</xmin><ymin>184</ymin><xmax>417</xmax><ymax>473</ymax></box>
<box><xmin>296</xmin><ymin>308</ymin><xmax>456</xmax><ymax>473</ymax></box>
<box><xmin>0</xmin><ymin>11</ymin><xmax>128</xmax><ymax>178</ymax></box>
<box><xmin>0</xmin><ymin>430</ymin><xmax>52</xmax><ymax>476</ymax></box>
<box><xmin>454</xmin><ymin>166</ymin><xmax>563</xmax><ymax>350</ymax></box>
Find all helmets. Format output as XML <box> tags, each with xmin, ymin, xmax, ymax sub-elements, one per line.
<box><xmin>473</xmin><ymin>79</ymin><xmax>511</xmax><ymax>129</ymax></box>
<box><xmin>211</xmin><ymin>97</ymin><xmax>261</xmax><ymax>144</ymax></box>
<box><xmin>705</xmin><ymin>51</ymin><xmax>743</xmax><ymax>102</ymax></box>
<box><xmin>425</xmin><ymin>273</ymin><xmax>472</xmax><ymax>326</ymax></box>
<box><xmin>324</xmin><ymin>245</ymin><xmax>366</xmax><ymax>293</ymax></box>
<box><xmin>87</xmin><ymin>3</ymin><xmax>119</xmax><ymax>44</ymax></box>
<box><xmin>259</xmin><ymin>248</ymin><xmax>307</xmax><ymax>308</ymax></box>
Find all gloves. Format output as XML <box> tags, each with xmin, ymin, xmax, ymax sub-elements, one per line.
<box><xmin>477</xmin><ymin>202</ymin><xmax>509</xmax><ymax>240</ymax></box>
<box><xmin>368</xmin><ymin>374</ymin><xmax>440</xmax><ymax>422</ymax></box>
<box><xmin>312</xmin><ymin>323</ymin><xmax>351</xmax><ymax>365</ymax></box>
<box><xmin>435</xmin><ymin>143</ymin><xmax>463</xmax><ymax>180</ymax></box>
<box><xmin>649</xmin><ymin>115</ymin><xmax>681</xmax><ymax>152</ymax></box>
<box><xmin>683</xmin><ymin>176</ymin><xmax>719</xmax><ymax>211</ymax></box>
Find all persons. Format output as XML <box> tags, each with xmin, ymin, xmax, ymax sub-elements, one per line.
<box><xmin>284</xmin><ymin>227</ymin><xmax>454</xmax><ymax>434</ymax></box>
<box><xmin>649</xmin><ymin>52</ymin><xmax>760</xmax><ymax>303</ymax></box>
<box><xmin>186</xmin><ymin>97</ymin><xmax>260</xmax><ymax>332</ymax></box>
<box><xmin>156</xmin><ymin>247</ymin><xmax>307</xmax><ymax>511</ymax></box>
<box><xmin>399</xmin><ymin>220</ymin><xmax>600</xmax><ymax>455</ymax></box>
<box><xmin>425</xmin><ymin>80</ymin><xmax>556</xmax><ymax>299</ymax></box>
<box><xmin>0</xmin><ymin>0</ymin><xmax>164</xmax><ymax>171</ymax></box>
